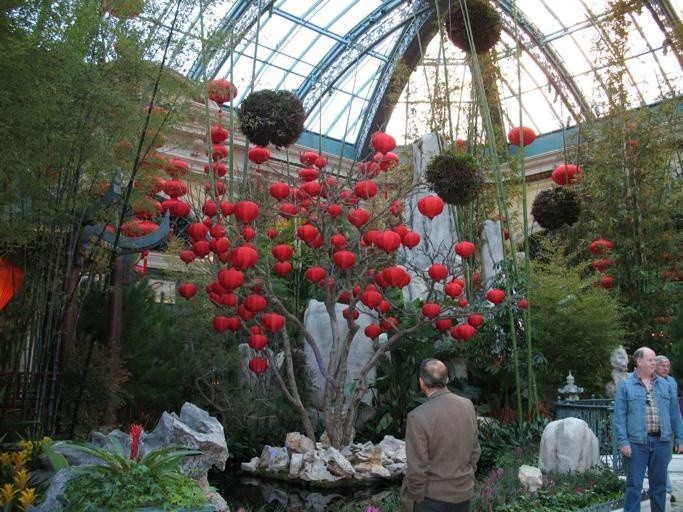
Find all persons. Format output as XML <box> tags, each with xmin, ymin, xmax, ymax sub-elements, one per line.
<box><xmin>655</xmin><ymin>355</ymin><xmax>679</xmax><ymax>505</ymax></box>
<box><xmin>613</xmin><ymin>346</ymin><xmax>682</xmax><ymax>512</ymax></box>
<box><xmin>405</xmin><ymin>358</ymin><xmax>483</xmax><ymax>512</ymax></box>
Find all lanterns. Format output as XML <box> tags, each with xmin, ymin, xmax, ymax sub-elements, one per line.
<box><xmin>93</xmin><ymin>78</ymin><xmax>538</xmax><ymax>378</ymax></box>
<box><xmin>552</xmin><ymin>165</ymin><xmax>583</xmax><ymax>186</ymax></box>
<box><xmin>589</xmin><ymin>239</ymin><xmax>618</xmax><ymax>289</ymax></box>
